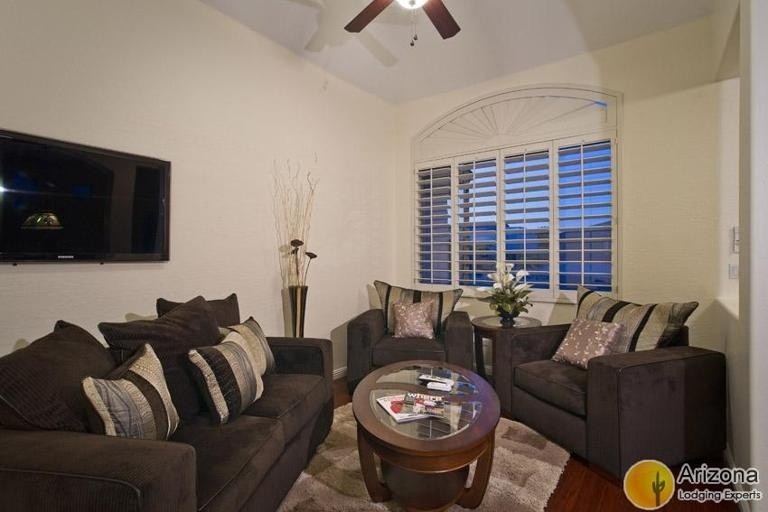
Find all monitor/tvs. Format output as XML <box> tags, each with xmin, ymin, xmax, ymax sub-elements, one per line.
<box><xmin>0</xmin><ymin>127</ymin><xmax>172</xmax><ymax>266</ymax></box>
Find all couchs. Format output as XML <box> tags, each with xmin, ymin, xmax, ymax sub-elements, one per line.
<box><xmin>346</xmin><ymin>308</ymin><xmax>473</xmax><ymax>396</ymax></box>
<box><xmin>0</xmin><ymin>300</ymin><xmax>334</xmax><ymax>512</ymax></box>
<box><xmin>495</xmin><ymin>323</ymin><xmax>727</xmax><ymax>482</ymax></box>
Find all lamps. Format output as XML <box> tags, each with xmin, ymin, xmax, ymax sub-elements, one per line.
<box><xmin>394</xmin><ymin>0</ymin><xmax>431</xmax><ymax>51</ymax></box>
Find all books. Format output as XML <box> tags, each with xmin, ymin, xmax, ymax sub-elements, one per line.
<box><xmin>376</xmin><ymin>391</ymin><xmax>446</xmax><ymax>424</ymax></box>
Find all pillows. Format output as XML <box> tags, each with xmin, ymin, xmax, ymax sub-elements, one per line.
<box><xmin>84</xmin><ymin>342</ymin><xmax>188</xmax><ymax>442</ymax></box>
<box><xmin>0</xmin><ymin>320</ymin><xmax>114</xmax><ymax>431</ymax></box>
<box><xmin>576</xmin><ymin>285</ymin><xmax>698</xmax><ymax>351</ymax></box>
<box><xmin>217</xmin><ymin>316</ymin><xmax>275</xmax><ymax>370</ymax></box>
<box><xmin>184</xmin><ymin>331</ymin><xmax>264</xmax><ymax>426</ymax></box>
<box><xmin>553</xmin><ymin>318</ymin><xmax>623</xmax><ymax>371</ymax></box>
<box><xmin>393</xmin><ymin>301</ymin><xmax>435</xmax><ymax>340</ymax></box>
<box><xmin>156</xmin><ymin>293</ymin><xmax>239</xmax><ymax>345</ymax></box>
<box><xmin>375</xmin><ymin>280</ymin><xmax>463</xmax><ymax>333</ymax></box>
<box><xmin>99</xmin><ymin>295</ymin><xmax>222</xmax><ymax>416</ymax></box>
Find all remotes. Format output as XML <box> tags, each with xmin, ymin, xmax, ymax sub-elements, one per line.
<box><xmin>426</xmin><ymin>381</ymin><xmax>451</xmax><ymax>392</ymax></box>
<box><xmin>418</xmin><ymin>373</ymin><xmax>455</xmax><ymax>387</ymax></box>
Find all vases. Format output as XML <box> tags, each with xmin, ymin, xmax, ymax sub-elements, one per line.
<box><xmin>499</xmin><ymin>312</ymin><xmax>518</xmax><ymax>327</ymax></box>
<box><xmin>289</xmin><ymin>285</ymin><xmax>308</xmax><ymax>337</ymax></box>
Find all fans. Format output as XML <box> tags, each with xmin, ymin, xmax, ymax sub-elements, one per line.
<box><xmin>343</xmin><ymin>0</ymin><xmax>460</xmax><ymax>56</ymax></box>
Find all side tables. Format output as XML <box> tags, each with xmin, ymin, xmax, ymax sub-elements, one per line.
<box><xmin>471</xmin><ymin>315</ymin><xmax>541</xmax><ymax>369</ymax></box>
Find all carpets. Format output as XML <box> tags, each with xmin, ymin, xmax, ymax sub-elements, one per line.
<box><xmin>275</xmin><ymin>401</ymin><xmax>571</xmax><ymax>511</ymax></box>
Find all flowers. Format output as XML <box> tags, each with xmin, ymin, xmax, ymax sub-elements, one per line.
<box><xmin>271</xmin><ymin>155</ymin><xmax>321</xmax><ymax>286</ymax></box>
<box><xmin>477</xmin><ymin>263</ymin><xmax>534</xmax><ymax>313</ymax></box>
